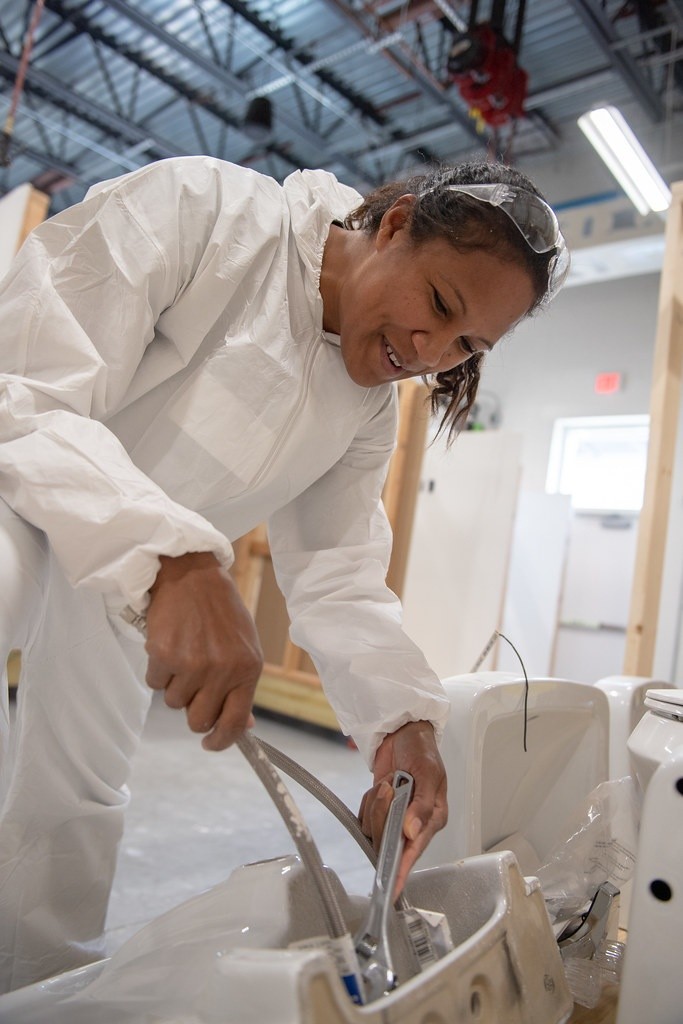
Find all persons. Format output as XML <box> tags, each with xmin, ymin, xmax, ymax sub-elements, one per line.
<box><xmin>0</xmin><ymin>160</ymin><xmax>564</xmax><ymax>999</ymax></box>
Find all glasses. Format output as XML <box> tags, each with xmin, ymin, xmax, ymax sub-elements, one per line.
<box><xmin>418</xmin><ymin>183</ymin><xmax>571</xmax><ymax>304</ymax></box>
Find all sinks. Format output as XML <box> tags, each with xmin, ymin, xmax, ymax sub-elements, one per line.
<box><xmin>433</xmin><ymin>671</ymin><xmax>614</xmax><ymax>943</ymax></box>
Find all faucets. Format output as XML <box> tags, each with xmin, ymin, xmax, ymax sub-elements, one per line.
<box><xmin>560</xmin><ymin>878</ymin><xmax>621</xmax><ymax>1006</ymax></box>
